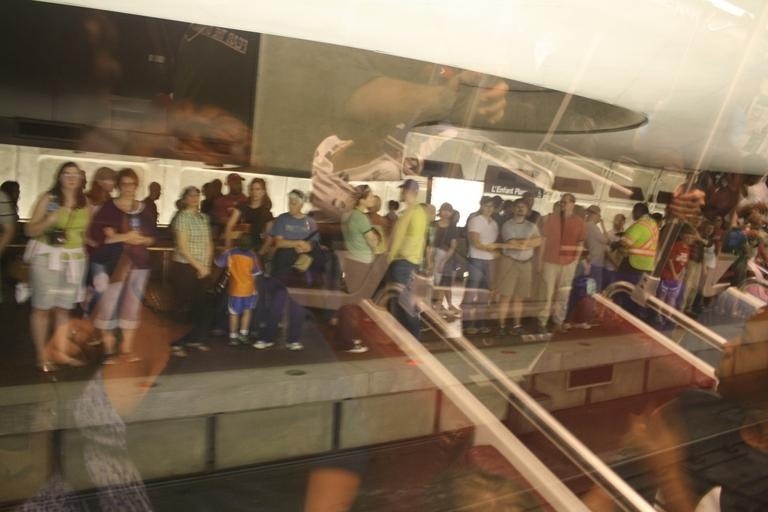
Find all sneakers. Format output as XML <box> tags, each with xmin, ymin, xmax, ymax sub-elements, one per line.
<box><xmin>252</xmin><ymin>339</ymin><xmax>275</xmax><ymax>349</ymax></box>
<box><xmin>100</xmin><ymin>353</ymin><xmax>117</xmax><ymax>366</ymax></box>
<box><xmin>462</xmin><ymin>326</ymin><xmax>480</xmax><ymax>336</ymax></box>
<box><xmin>228</xmin><ymin>336</ymin><xmax>241</xmax><ymax>346</ymax></box>
<box><xmin>479</xmin><ymin>325</ymin><xmax>492</xmax><ymax>334</ymax></box>
<box><xmin>286</xmin><ymin>341</ymin><xmax>304</xmax><ymax>351</ymax></box>
<box><xmin>119</xmin><ymin>352</ymin><xmax>142</xmax><ymax>364</ymax></box>
<box><xmin>509</xmin><ymin>325</ymin><xmax>531</xmax><ymax>337</ymax></box>
<box><xmin>239</xmin><ymin>333</ymin><xmax>251</xmax><ymax>345</ymax></box>
<box><xmin>344</xmin><ymin>339</ymin><xmax>369</xmax><ymax>354</ymax></box>
<box><xmin>498</xmin><ymin>327</ymin><xmax>508</xmax><ymax>338</ymax></box>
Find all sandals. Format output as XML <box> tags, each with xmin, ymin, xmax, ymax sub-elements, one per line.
<box><xmin>47</xmin><ymin>354</ymin><xmax>86</xmax><ymax>368</ymax></box>
<box><xmin>35</xmin><ymin>360</ymin><xmax>57</xmax><ymax>374</ymax></box>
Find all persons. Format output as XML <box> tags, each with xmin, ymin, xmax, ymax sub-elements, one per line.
<box><xmin>4</xmin><ymin>61</ymin><xmax>255</xmax><ymax>151</ymax></box>
<box><xmin>0</xmin><ymin>173</ymin><xmax>767</xmax><ymax>512</ymax></box>
<box><xmin>261</xmin><ymin>55</ymin><xmax>508</xmax><ymax>172</ymax></box>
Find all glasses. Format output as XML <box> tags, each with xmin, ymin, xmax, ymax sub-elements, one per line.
<box><xmin>62</xmin><ymin>170</ymin><xmax>81</xmax><ymax>179</ymax></box>
<box><xmin>122</xmin><ymin>182</ymin><xmax>137</xmax><ymax>188</ymax></box>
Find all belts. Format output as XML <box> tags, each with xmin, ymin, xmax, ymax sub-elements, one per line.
<box><xmin>506</xmin><ymin>255</ymin><xmax>533</xmax><ymax>264</ymax></box>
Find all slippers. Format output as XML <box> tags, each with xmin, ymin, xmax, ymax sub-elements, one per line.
<box><xmin>185</xmin><ymin>340</ymin><xmax>213</xmax><ymax>355</ymax></box>
<box><xmin>168</xmin><ymin>347</ymin><xmax>188</xmax><ymax>360</ymax></box>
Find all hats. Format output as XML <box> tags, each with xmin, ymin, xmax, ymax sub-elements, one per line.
<box><xmin>228</xmin><ymin>173</ymin><xmax>245</xmax><ymax>183</ymax></box>
<box><xmin>396</xmin><ymin>179</ymin><xmax>419</xmax><ymax>192</ymax></box>
<box><xmin>439</xmin><ymin>202</ymin><xmax>453</xmax><ymax>212</ymax></box>
<box><xmin>560</xmin><ymin>193</ymin><xmax>576</xmax><ymax>202</ymax></box>
